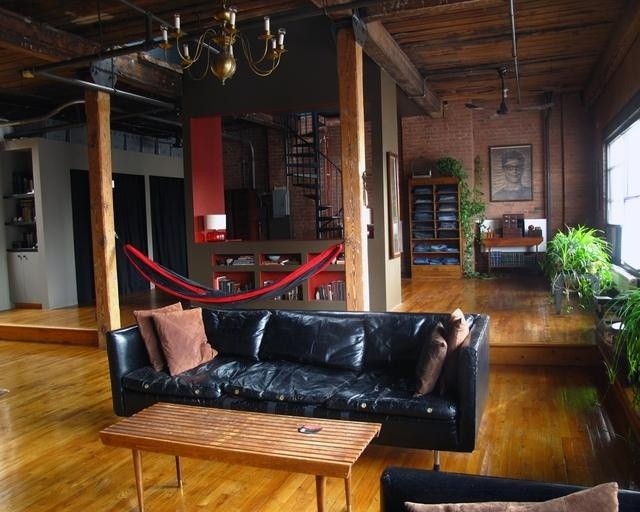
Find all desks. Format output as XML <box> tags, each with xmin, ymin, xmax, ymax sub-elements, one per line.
<box><xmin>482</xmin><ymin>236</ymin><xmax>543</xmax><ymax>275</ymax></box>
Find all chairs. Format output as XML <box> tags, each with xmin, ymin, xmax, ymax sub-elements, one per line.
<box><xmin>380</xmin><ymin>469</ymin><xmax>640</xmax><ymax>512</ymax></box>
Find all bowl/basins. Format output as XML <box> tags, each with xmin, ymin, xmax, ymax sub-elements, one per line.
<box><xmin>269</xmin><ymin>256</ymin><xmax>280</xmax><ymax>261</ymax></box>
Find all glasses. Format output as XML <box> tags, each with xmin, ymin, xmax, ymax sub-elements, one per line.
<box><xmin>504</xmin><ymin>164</ymin><xmax>522</xmax><ymax>170</ymax></box>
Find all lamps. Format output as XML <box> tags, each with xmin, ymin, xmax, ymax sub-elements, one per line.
<box><xmin>202</xmin><ymin>214</ymin><xmax>228</xmax><ymax>243</ymax></box>
<box><xmin>158</xmin><ymin>0</ymin><xmax>288</xmax><ymax>87</ymax></box>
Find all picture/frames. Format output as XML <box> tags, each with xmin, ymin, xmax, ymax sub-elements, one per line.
<box><xmin>386</xmin><ymin>150</ymin><xmax>405</xmax><ymax>259</ymax></box>
<box><xmin>489</xmin><ymin>143</ymin><xmax>534</xmax><ymax>203</ymax></box>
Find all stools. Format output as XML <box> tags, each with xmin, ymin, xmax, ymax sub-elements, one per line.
<box><xmin>554</xmin><ymin>272</ymin><xmax>599</xmax><ymax>314</ymax></box>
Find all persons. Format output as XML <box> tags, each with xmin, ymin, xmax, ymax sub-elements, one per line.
<box><xmin>492</xmin><ymin>149</ymin><xmax>531</xmax><ymax>200</ymax></box>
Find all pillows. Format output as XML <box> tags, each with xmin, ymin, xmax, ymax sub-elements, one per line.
<box><xmin>403</xmin><ymin>481</ymin><xmax>619</xmax><ymax>512</ymax></box>
<box><xmin>445</xmin><ymin>308</ymin><xmax>470</xmax><ymax>352</ymax></box>
<box><xmin>191</xmin><ymin>304</ymin><xmax>271</xmax><ymax>365</ymax></box>
<box><xmin>133</xmin><ymin>301</ymin><xmax>182</xmax><ymax>371</ymax></box>
<box><xmin>363</xmin><ymin>314</ymin><xmax>449</xmax><ymax>376</ymax></box>
<box><xmin>413</xmin><ymin>322</ymin><xmax>448</xmax><ymax>399</ymax></box>
<box><xmin>260</xmin><ymin>310</ymin><xmax>367</xmax><ymax>371</ymax></box>
<box><xmin>151</xmin><ymin>308</ymin><xmax>219</xmax><ymax>376</ymax></box>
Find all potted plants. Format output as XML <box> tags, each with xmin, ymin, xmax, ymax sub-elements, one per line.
<box><xmin>601</xmin><ymin>289</ymin><xmax>640</xmax><ymax>410</ymax></box>
<box><xmin>436</xmin><ymin>156</ymin><xmax>488</xmax><ymax>280</ymax></box>
<box><xmin>544</xmin><ymin>224</ymin><xmax>615</xmax><ymax>315</ymax></box>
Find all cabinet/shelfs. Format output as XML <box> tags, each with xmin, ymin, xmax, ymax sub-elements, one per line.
<box><xmin>502</xmin><ymin>214</ymin><xmax>524</xmax><ymax>237</ymax></box>
<box><xmin>0</xmin><ymin>148</ymin><xmax>79</xmax><ymax>310</ymax></box>
<box><xmin>408</xmin><ymin>176</ymin><xmax>462</xmax><ymax>281</ymax></box>
<box><xmin>210</xmin><ymin>239</ymin><xmax>346</xmax><ymax>311</ymax></box>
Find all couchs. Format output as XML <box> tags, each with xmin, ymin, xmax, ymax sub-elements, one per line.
<box><xmin>106</xmin><ymin>301</ymin><xmax>491</xmax><ymax>472</ymax></box>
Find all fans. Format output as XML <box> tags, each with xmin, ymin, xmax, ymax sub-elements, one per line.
<box><xmin>465</xmin><ymin>67</ymin><xmax>554</xmax><ymax>122</ymax></box>
